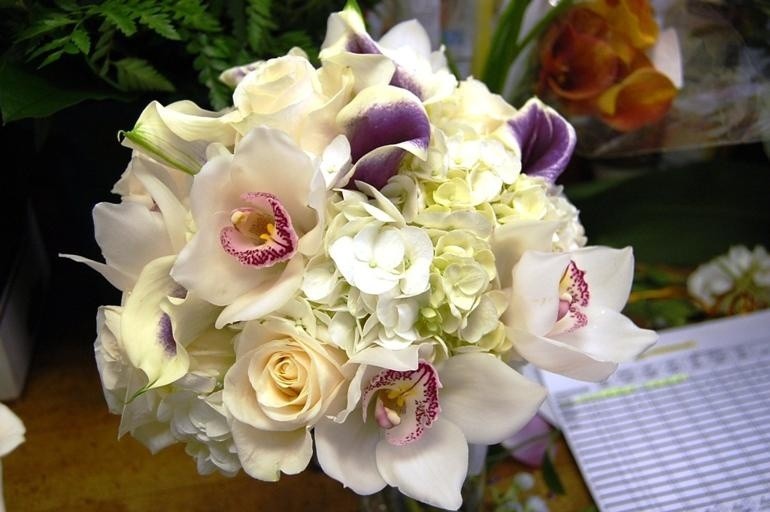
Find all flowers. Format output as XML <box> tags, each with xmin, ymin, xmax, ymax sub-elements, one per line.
<box><xmin>56</xmin><ymin>0</ymin><xmax>661</xmax><ymax>512</ymax></box>
<box><xmin>439</xmin><ymin>0</ymin><xmax>683</xmax><ymax>136</ymax></box>
<box><xmin>683</xmin><ymin>242</ymin><xmax>769</xmax><ymax>317</ymax></box>
